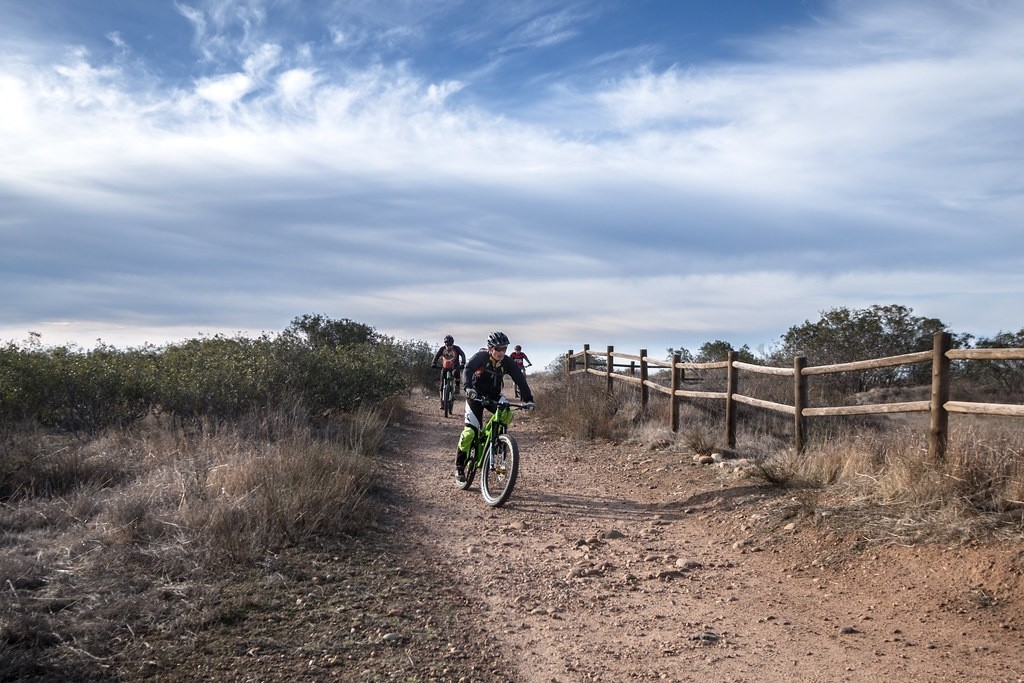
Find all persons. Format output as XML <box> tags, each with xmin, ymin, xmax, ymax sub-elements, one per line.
<box><xmin>455</xmin><ymin>331</ymin><xmax>534</xmax><ymax>487</ymax></box>
<box><xmin>430</xmin><ymin>335</ymin><xmax>466</xmax><ymax>410</ymax></box>
<box><xmin>509</xmin><ymin>344</ymin><xmax>531</xmax><ymax>398</ymax></box>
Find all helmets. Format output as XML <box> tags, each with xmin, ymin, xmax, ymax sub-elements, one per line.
<box><xmin>514</xmin><ymin>345</ymin><xmax>521</xmax><ymax>350</ymax></box>
<box><xmin>487</xmin><ymin>332</ymin><xmax>510</xmax><ymax>347</ymax></box>
<box><xmin>444</xmin><ymin>335</ymin><xmax>454</xmax><ymax>342</ymax></box>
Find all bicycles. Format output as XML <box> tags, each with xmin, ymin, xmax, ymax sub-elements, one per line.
<box><xmin>515</xmin><ymin>364</ymin><xmax>531</xmax><ymax>395</ymax></box>
<box><xmin>455</xmin><ymin>393</ymin><xmax>536</xmax><ymax>508</ymax></box>
<box><xmin>434</xmin><ymin>364</ymin><xmax>465</xmax><ymax>416</ymax></box>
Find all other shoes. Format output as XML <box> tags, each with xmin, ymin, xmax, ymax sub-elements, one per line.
<box><xmin>455</xmin><ymin>464</ymin><xmax>465</xmax><ymax>482</ymax></box>
<box><xmin>455</xmin><ymin>384</ymin><xmax>460</xmax><ymax>394</ymax></box>
<box><xmin>439</xmin><ymin>401</ymin><xmax>444</xmax><ymax>410</ymax></box>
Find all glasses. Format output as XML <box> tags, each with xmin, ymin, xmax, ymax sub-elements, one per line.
<box><xmin>517</xmin><ymin>349</ymin><xmax>521</xmax><ymax>351</ymax></box>
<box><xmin>445</xmin><ymin>342</ymin><xmax>453</xmax><ymax>346</ymax></box>
<box><xmin>493</xmin><ymin>346</ymin><xmax>507</xmax><ymax>352</ymax></box>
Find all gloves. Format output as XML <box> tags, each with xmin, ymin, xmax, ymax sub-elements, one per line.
<box><xmin>465</xmin><ymin>387</ymin><xmax>477</xmax><ymax>399</ymax></box>
<box><xmin>527</xmin><ymin>401</ymin><xmax>535</xmax><ymax>411</ymax></box>
<box><xmin>431</xmin><ymin>362</ymin><xmax>437</xmax><ymax>369</ymax></box>
<box><xmin>459</xmin><ymin>365</ymin><xmax>464</xmax><ymax>370</ymax></box>
